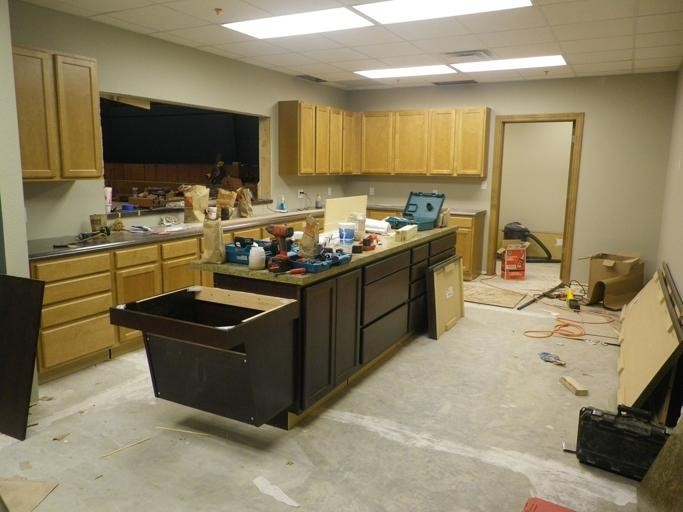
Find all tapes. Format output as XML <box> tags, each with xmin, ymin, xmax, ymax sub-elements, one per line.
<box><xmin>122</xmin><ymin>205</ymin><xmax>135</xmax><ymax>211</ymax></box>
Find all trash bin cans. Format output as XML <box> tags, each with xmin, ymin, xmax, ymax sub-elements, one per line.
<box><xmin>504</xmin><ymin>222</ymin><xmax>529</xmax><ymax>243</ymax></box>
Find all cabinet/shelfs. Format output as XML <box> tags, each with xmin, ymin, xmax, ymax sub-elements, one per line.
<box><xmin>446</xmin><ymin>211</ymin><xmax>486</xmax><ymax>281</ymax></box>
<box><xmin>210</xmin><ymin>266</ymin><xmax>365</xmax><ymax>436</ymax></box>
<box><xmin>368</xmin><ymin>207</ymin><xmax>396</xmax><ymax>220</ymax></box>
<box><xmin>429</xmin><ymin>224</ymin><xmax>458</xmax><ymax>266</ymax></box>
<box><xmin>199</xmin><ymin>222</ymin><xmax>235</xmax><ymax>289</ymax></box>
<box><xmin>278</xmin><ymin>96</ymin><xmax>493</xmax><ymax>181</ymax></box>
<box><xmin>110</xmin><ymin>235</ymin><xmax>167</xmax><ymax>359</ymax></box>
<box><xmin>356</xmin><ymin>244</ymin><xmax>410</xmax><ymax>380</ymax></box>
<box><xmin>309</xmin><ymin>209</ymin><xmax>325</xmax><ymax>232</ymax></box>
<box><xmin>409</xmin><ymin>235</ymin><xmax>431</xmax><ymax>340</ymax></box>
<box><xmin>161</xmin><ymin>231</ymin><xmax>203</xmax><ymax>292</ymax></box>
<box><xmin>397</xmin><ymin>208</ymin><xmax>402</xmax><ymax>217</ymax></box>
<box><xmin>284</xmin><ymin>213</ymin><xmax>310</xmax><ymax>233</ymax></box>
<box><xmin>260</xmin><ymin>217</ymin><xmax>286</xmax><ymax>241</ymax></box>
<box><xmin>10</xmin><ymin>36</ymin><xmax>105</xmax><ymax>186</ymax></box>
<box><xmin>230</xmin><ymin>220</ymin><xmax>262</xmax><ymax>241</ymax></box>
<box><xmin>30</xmin><ymin>248</ymin><xmax>123</xmax><ymax>387</ymax></box>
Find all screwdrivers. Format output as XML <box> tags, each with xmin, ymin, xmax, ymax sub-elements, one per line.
<box><xmin>275</xmin><ymin>268</ymin><xmax>306</xmax><ymax>276</ymax></box>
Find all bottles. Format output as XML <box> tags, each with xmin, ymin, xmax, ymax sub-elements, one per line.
<box><xmin>314</xmin><ymin>193</ymin><xmax>322</xmax><ymax>208</ymax></box>
<box><xmin>278</xmin><ymin>196</ymin><xmax>286</xmax><ymax>210</ymax></box>
<box><xmin>131</xmin><ymin>186</ymin><xmax>173</xmax><ymax>208</ymax></box>
<box><xmin>248</xmin><ymin>246</ymin><xmax>265</xmax><ymax>269</ymax></box>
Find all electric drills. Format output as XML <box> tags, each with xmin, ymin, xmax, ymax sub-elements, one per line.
<box><xmin>264</xmin><ymin>226</ymin><xmax>294</xmax><ymax>271</ymax></box>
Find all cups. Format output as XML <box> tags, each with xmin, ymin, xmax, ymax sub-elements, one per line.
<box><xmin>89</xmin><ymin>214</ymin><xmax>102</xmax><ymax>231</ymax></box>
<box><xmin>335</xmin><ymin>212</ymin><xmax>365</xmax><ymax>243</ymax></box>
<box><xmin>104</xmin><ymin>187</ymin><xmax>112</xmax><ymax>214</ymax></box>
<box><xmin>205</xmin><ymin>207</ymin><xmax>217</xmax><ymax>214</ymax></box>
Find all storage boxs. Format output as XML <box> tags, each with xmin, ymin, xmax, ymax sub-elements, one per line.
<box><xmin>578</xmin><ymin>251</ymin><xmax>646</xmax><ymax>312</ymax></box>
<box><xmin>494</xmin><ymin>238</ymin><xmax>531</xmax><ymax>281</ymax></box>
